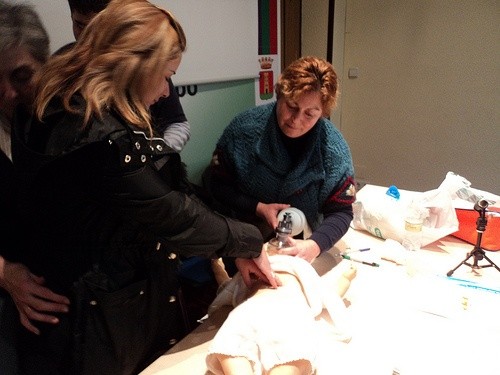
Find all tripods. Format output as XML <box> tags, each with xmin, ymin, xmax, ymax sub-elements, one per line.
<box><xmin>447</xmin><ymin>199</ymin><xmax>500</xmax><ymax>276</ymax></box>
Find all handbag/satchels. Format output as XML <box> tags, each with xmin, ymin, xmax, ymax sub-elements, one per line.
<box><xmin>349</xmin><ymin>184</ymin><xmax>459</xmax><ymax>247</ymax></box>
<box><xmin>70</xmin><ymin>264</ymin><xmax>187</xmax><ymax>375</ymax></box>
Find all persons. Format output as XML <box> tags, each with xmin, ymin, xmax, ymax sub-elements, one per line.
<box><xmin>25</xmin><ymin>0</ymin><xmax>265</xmax><ymax>375</ymax></box>
<box><xmin>51</xmin><ymin>1</ymin><xmax>192</xmax><ymax>154</ymax></box>
<box><xmin>195</xmin><ymin>55</ymin><xmax>357</xmax><ymax>263</ymax></box>
<box><xmin>202</xmin><ymin>236</ymin><xmax>357</xmax><ymax>375</ymax></box>
<box><xmin>0</xmin><ymin>0</ymin><xmax>77</xmax><ymax>374</ymax></box>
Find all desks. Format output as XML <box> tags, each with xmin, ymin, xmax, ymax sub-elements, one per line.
<box><xmin>138</xmin><ymin>184</ymin><xmax>500</xmax><ymax>375</ymax></box>
